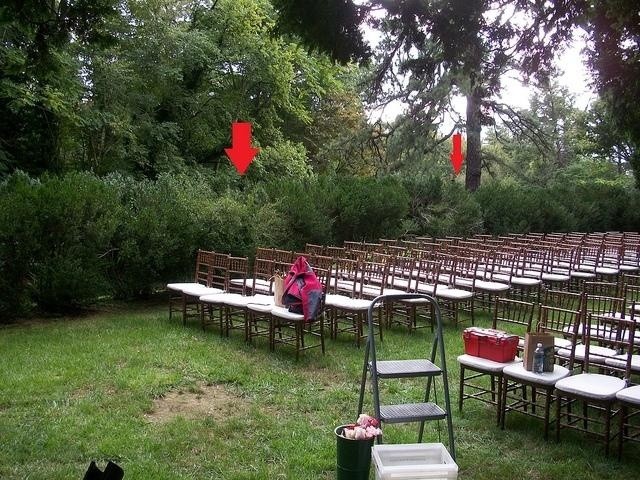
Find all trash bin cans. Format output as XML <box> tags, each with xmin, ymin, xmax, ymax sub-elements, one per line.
<box><xmin>334</xmin><ymin>424</ymin><xmax>375</xmax><ymax>480</ymax></box>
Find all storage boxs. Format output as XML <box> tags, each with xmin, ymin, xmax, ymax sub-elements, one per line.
<box><xmin>370</xmin><ymin>442</ymin><xmax>461</xmax><ymax>480</ymax></box>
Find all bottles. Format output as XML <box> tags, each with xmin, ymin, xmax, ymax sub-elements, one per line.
<box><xmin>532</xmin><ymin>343</ymin><xmax>544</xmax><ymax>374</ymax></box>
